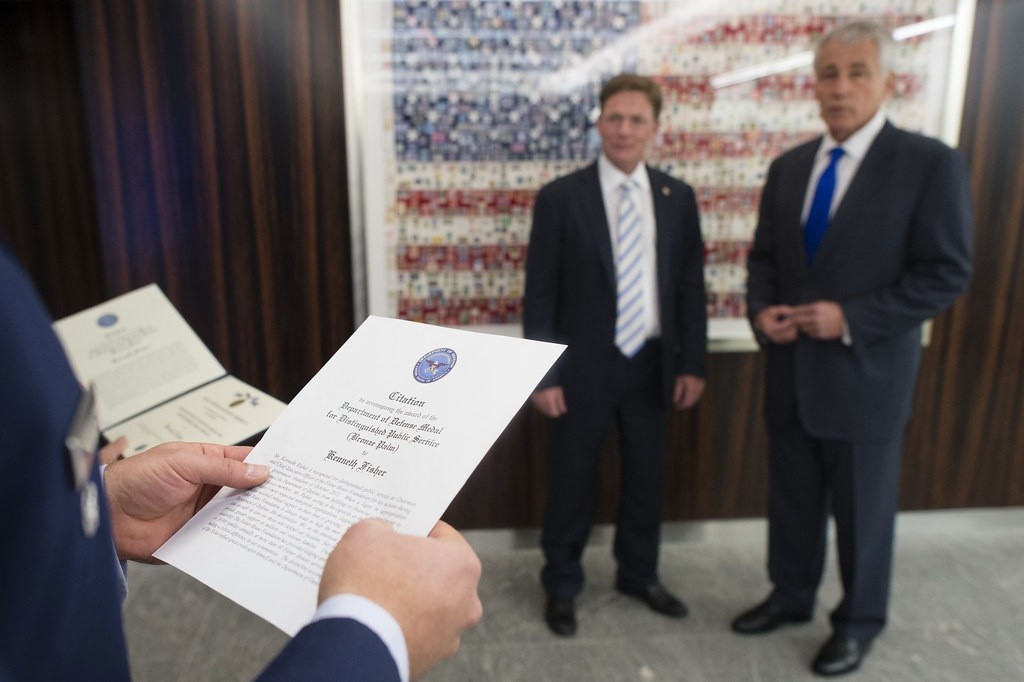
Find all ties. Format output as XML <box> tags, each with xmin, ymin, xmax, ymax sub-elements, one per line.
<box><xmin>614</xmin><ymin>182</ymin><xmax>646</xmax><ymax>358</ymax></box>
<box><xmin>802</xmin><ymin>148</ymin><xmax>844</xmax><ymax>267</ymax></box>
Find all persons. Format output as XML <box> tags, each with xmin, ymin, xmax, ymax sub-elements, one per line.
<box><xmin>731</xmin><ymin>24</ymin><xmax>972</xmax><ymax>680</ymax></box>
<box><xmin>521</xmin><ymin>73</ymin><xmax>708</xmax><ymax>636</ymax></box>
<box><xmin>0</xmin><ymin>231</ymin><xmax>482</xmax><ymax>682</ymax></box>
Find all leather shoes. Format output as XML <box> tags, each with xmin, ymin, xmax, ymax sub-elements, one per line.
<box><xmin>546</xmin><ymin>582</ymin><xmax>575</xmax><ymax>634</ymax></box>
<box><xmin>617</xmin><ymin>575</ymin><xmax>686</xmax><ymax>616</ymax></box>
<box><xmin>732</xmin><ymin>600</ymin><xmax>812</xmax><ymax>633</ymax></box>
<box><xmin>812</xmin><ymin>631</ymin><xmax>868</xmax><ymax>674</ymax></box>
